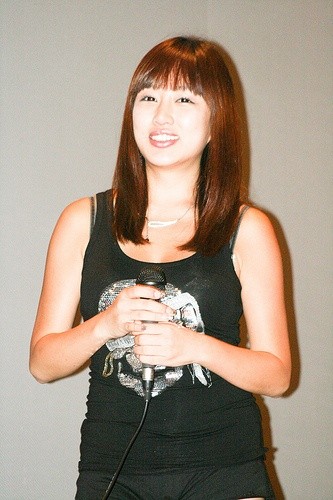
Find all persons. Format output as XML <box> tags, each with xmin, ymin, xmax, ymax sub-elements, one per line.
<box><xmin>29</xmin><ymin>36</ymin><xmax>292</xmax><ymax>500</ymax></box>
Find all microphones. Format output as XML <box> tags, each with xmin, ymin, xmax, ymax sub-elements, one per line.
<box><xmin>135</xmin><ymin>264</ymin><xmax>167</xmax><ymax>402</ymax></box>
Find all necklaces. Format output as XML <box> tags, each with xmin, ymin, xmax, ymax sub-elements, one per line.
<box><xmin>144</xmin><ymin>205</ymin><xmax>195</xmax><ymax>228</ymax></box>
